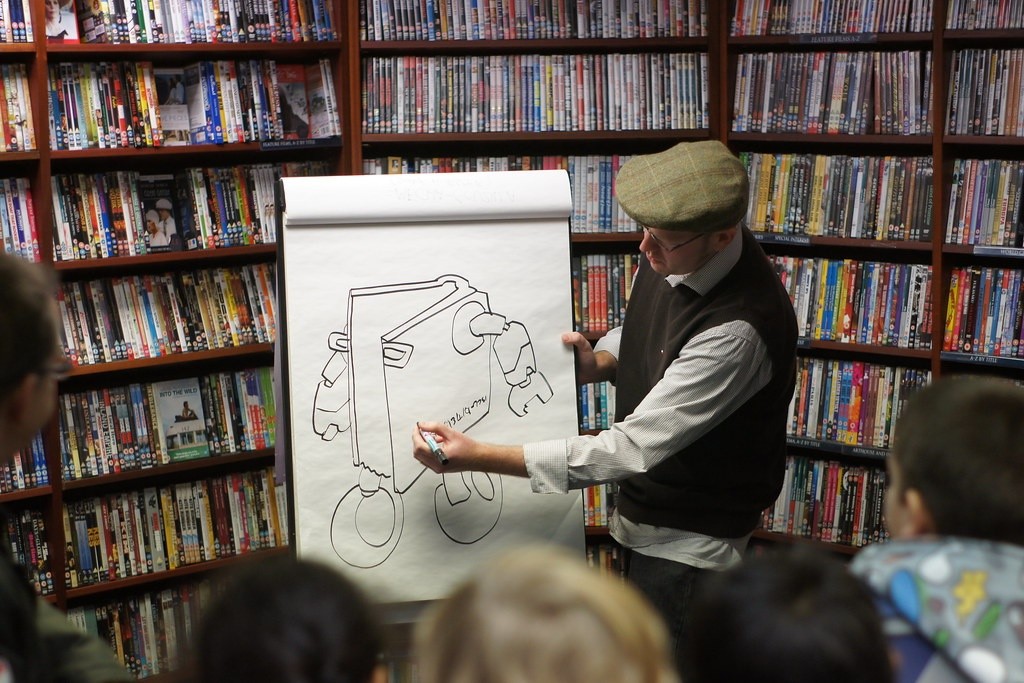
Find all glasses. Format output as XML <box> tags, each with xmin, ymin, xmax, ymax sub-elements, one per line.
<box><xmin>641</xmin><ymin>225</ymin><xmax>711</xmax><ymax>254</ymax></box>
<box><xmin>33</xmin><ymin>352</ymin><xmax>76</xmax><ymax>381</ymax></box>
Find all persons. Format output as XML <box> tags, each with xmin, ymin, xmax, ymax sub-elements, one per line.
<box><xmin>0</xmin><ymin>252</ymin><xmax>138</xmax><ymax>683</ymax></box>
<box><xmin>673</xmin><ymin>531</ymin><xmax>895</xmax><ymax>683</ymax></box>
<box><xmin>172</xmin><ymin>74</ymin><xmax>185</xmax><ymax>105</ymax></box>
<box><xmin>145</xmin><ymin>210</ymin><xmax>169</xmax><ymax>246</ymax></box>
<box><xmin>45</xmin><ymin>0</ymin><xmax>69</xmax><ymax>40</ymax></box>
<box><xmin>844</xmin><ymin>376</ymin><xmax>1024</xmax><ymax>683</ymax></box>
<box><xmin>189</xmin><ymin>558</ymin><xmax>393</xmax><ymax>683</ymax></box>
<box><xmin>411</xmin><ymin>546</ymin><xmax>679</xmax><ymax>683</ymax></box>
<box><xmin>164</xmin><ymin>78</ymin><xmax>176</xmax><ymax>105</ymax></box>
<box><xmin>412</xmin><ymin>141</ymin><xmax>798</xmax><ymax>658</ymax></box>
<box><xmin>182</xmin><ymin>401</ymin><xmax>195</xmax><ymax>417</ymax></box>
<box><xmin>156</xmin><ymin>200</ymin><xmax>176</xmax><ymax>242</ymax></box>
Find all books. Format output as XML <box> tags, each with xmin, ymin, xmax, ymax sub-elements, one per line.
<box><xmin>0</xmin><ymin>0</ymin><xmax>1024</xmax><ymax>683</ymax></box>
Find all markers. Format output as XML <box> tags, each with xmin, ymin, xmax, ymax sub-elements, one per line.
<box><xmin>413</xmin><ymin>421</ymin><xmax>450</xmax><ymax>467</ymax></box>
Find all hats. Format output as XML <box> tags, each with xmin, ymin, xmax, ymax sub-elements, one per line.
<box><xmin>614</xmin><ymin>139</ymin><xmax>750</xmax><ymax>233</ymax></box>
